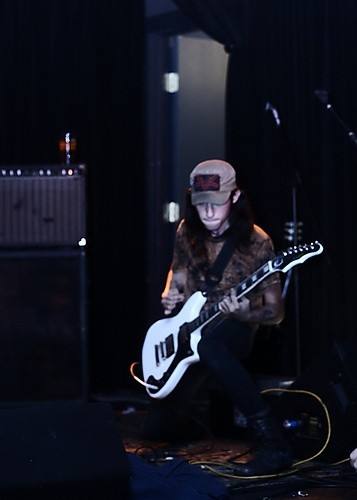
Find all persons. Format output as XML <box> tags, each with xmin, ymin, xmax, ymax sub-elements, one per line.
<box><xmin>141</xmin><ymin>159</ymin><xmax>290</xmax><ymax>476</ymax></box>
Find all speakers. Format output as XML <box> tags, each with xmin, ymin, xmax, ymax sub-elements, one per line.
<box><xmin>0</xmin><ymin>401</ymin><xmax>132</xmax><ymax>500</ymax></box>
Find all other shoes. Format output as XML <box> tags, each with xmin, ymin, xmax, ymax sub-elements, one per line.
<box><xmin>238</xmin><ymin>407</ymin><xmax>299</xmax><ymax>466</ymax></box>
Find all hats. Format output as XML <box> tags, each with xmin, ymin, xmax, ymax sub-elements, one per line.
<box><xmin>189</xmin><ymin>160</ymin><xmax>238</xmax><ymax>205</ymax></box>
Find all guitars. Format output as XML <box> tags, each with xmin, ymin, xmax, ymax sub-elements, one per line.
<box><xmin>141</xmin><ymin>240</ymin><xmax>323</xmax><ymax>398</ymax></box>
